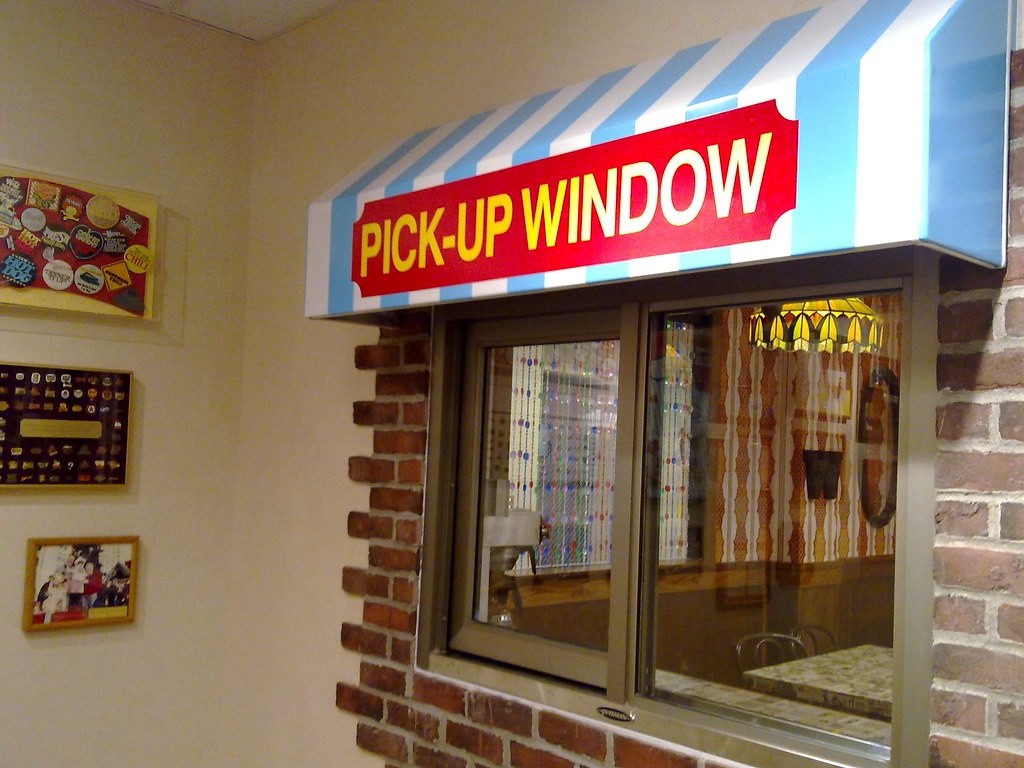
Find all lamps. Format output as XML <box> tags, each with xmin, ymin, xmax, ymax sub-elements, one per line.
<box><xmin>748</xmin><ymin>297</ymin><xmax>884</xmax><ymax>355</ymax></box>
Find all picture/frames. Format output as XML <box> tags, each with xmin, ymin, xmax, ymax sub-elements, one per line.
<box><xmin>21</xmin><ymin>536</ymin><xmax>139</xmax><ymax>631</ymax></box>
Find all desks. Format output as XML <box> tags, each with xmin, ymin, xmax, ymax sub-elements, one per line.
<box><xmin>744</xmin><ymin>648</ymin><xmax>893</xmax><ymax>721</ymax></box>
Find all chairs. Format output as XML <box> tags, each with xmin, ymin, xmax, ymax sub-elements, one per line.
<box><xmin>735</xmin><ymin>624</ymin><xmax>836</xmax><ymax>693</ymax></box>
